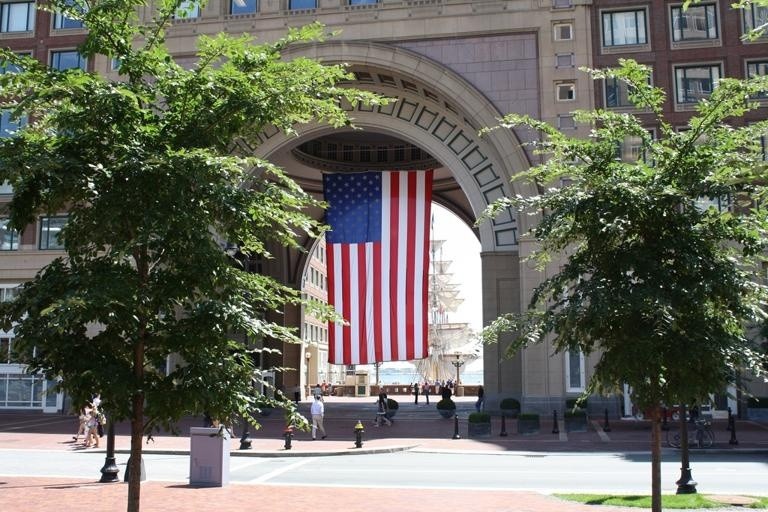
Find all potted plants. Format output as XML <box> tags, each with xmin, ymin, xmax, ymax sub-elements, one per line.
<box><xmin>436</xmin><ymin>399</ymin><xmax>456</xmax><ymax>418</ymax></box>
<box><xmin>518</xmin><ymin>413</ymin><xmax>540</xmax><ymax>436</ymax></box>
<box><xmin>500</xmin><ymin>399</ymin><xmax>519</xmax><ymax>417</ymax></box>
<box><xmin>381</xmin><ymin>399</ymin><xmax>398</xmax><ymax>419</ymax></box>
<box><xmin>468</xmin><ymin>412</ymin><xmax>491</xmax><ymax>439</ymax></box>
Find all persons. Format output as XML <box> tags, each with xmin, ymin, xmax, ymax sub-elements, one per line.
<box><xmin>72</xmin><ymin>392</ymin><xmax>107</xmax><ymax>449</ymax></box>
<box><xmin>688</xmin><ymin>398</ymin><xmax>700</xmax><ymax>444</ymax></box>
<box><xmin>408</xmin><ymin>376</ymin><xmax>462</xmax><ymax>405</ymax></box>
<box><xmin>372</xmin><ymin>387</ymin><xmax>399</xmax><ymax>428</ymax></box>
<box><xmin>210</xmin><ymin>419</ymin><xmax>225</xmax><ymax>428</ymax></box>
<box><xmin>294</xmin><ymin>384</ymin><xmax>300</xmax><ymax>404</ymax></box>
<box><xmin>310</xmin><ymin>395</ymin><xmax>327</xmax><ymax>440</ymax></box>
<box><xmin>475</xmin><ymin>385</ymin><xmax>484</xmax><ymax>413</ymax></box>
<box><xmin>313</xmin><ymin>384</ymin><xmax>321</xmax><ymax>401</ymax></box>
<box><xmin>328</xmin><ymin>382</ymin><xmax>332</xmax><ymax>393</ymax></box>
<box><xmin>321</xmin><ymin>379</ymin><xmax>328</xmax><ymax>394</ymax></box>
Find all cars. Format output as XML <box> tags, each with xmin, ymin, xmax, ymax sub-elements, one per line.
<box><xmin>632</xmin><ymin>400</ymin><xmax>681</xmax><ymax>423</ymax></box>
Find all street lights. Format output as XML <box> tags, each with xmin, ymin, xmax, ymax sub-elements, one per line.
<box><xmin>305</xmin><ymin>351</ymin><xmax>311</xmax><ymax>397</ymax></box>
<box><xmin>451</xmin><ymin>351</ymin><xmax>465</xmax><ymax>386</ymax></box>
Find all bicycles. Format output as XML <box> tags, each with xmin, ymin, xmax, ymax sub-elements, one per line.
<box><xmin>668</xmin><ymin>417</ymin><xmax>715</xmax><ymax>450</ymax></box>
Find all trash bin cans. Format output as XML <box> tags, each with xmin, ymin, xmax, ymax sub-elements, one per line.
<box><xmin>337</xmin><ymin>387</ymin><xmax>343</xmax><ymax>396</ymax></box>
<box><xmin>190</xmin><ymin>427</ymin><xmax>231</xmax><ymax>488</ymax></box>
<box><xmin>457</xmin><ymin>384</ymin><xmax>465</xmax><ymax>396</ymax></box>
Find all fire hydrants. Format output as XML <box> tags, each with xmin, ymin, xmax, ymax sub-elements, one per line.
<box><xmin>352</xmin><ymin>420</ymin><xmax>365</xmax><ymax>448</ymax></box>
<box><xmin>283</xmin><ymin>424</ymin><xmax>294</xmax><ymax>449</ymax></box>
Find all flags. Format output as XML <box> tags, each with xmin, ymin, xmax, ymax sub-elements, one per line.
<box><xmin>320</xmin><ymin>168</ymin><xmax>436</xmax><ymax>366</ymax></box>
